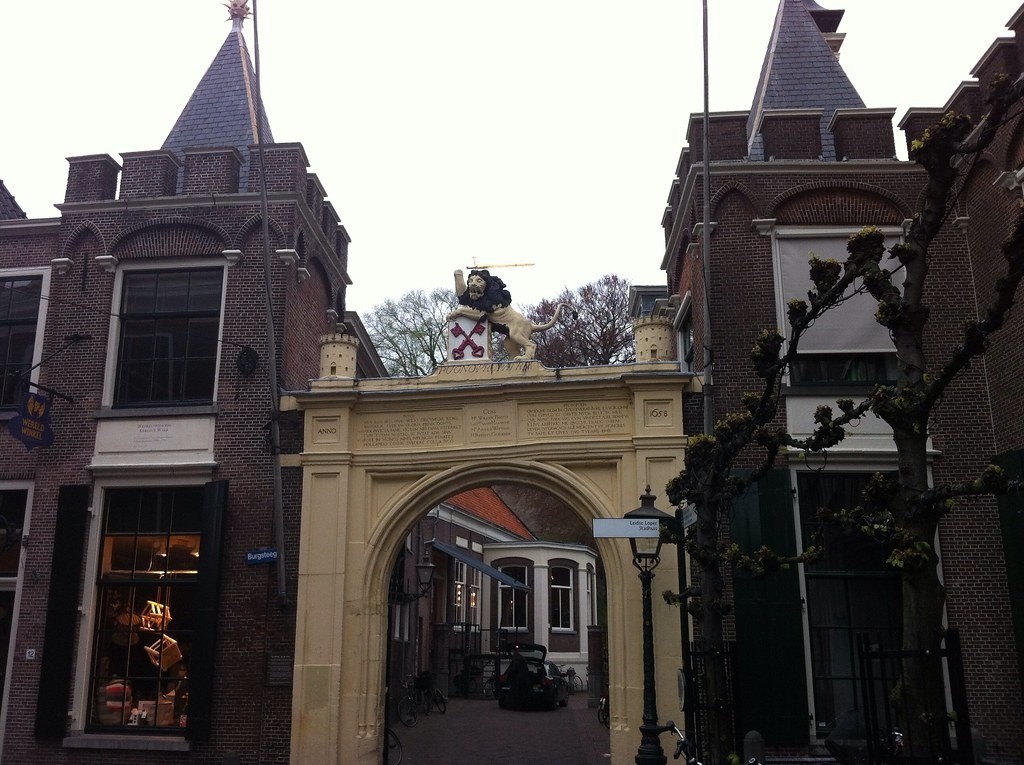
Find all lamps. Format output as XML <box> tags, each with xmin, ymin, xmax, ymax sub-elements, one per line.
<box><xmin>145</xmin><ymin>538</ymin><xmax>168</xmax><ymax>572</ymax></box>
<box><xmin>414</xmin><ymin>547</ymin><xmax>436</xmax><ymax>599</ymax></box>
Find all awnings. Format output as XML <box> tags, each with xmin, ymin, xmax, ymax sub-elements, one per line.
<box><xmin>434</xmin><ymin>540</ymin><xmax>532</xmax><ymax>594</ymax></box>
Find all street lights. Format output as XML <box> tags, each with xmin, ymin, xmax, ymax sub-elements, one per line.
<box><xmin>622</xmin><ymin>483</ymin><xmax>677</xmax><ymax>764</ymax></box>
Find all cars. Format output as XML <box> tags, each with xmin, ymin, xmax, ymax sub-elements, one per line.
<box><xmin>498</xmin><ymin>657</ymin><xmax>570</xmax><ymax>712</ymax></box>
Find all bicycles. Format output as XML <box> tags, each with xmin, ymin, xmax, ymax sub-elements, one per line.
<box><xmin>396</xmin><ymin>673</ymin><xmax>419</xmax><ymax>726</ymax></box>
<box><xmin>660</xmin><ymin>717</ymin><xmax>766</xmax><ymax>765</ymax></box>
<box><xmin>597</xmin><ymin>684</ymin><xmax>610</xmax><ymax>729</ymax></box>
<box><xmin>453</xmin><ymin>648</ymin><xmax>584</xmax><ymax>698</ymax></box>
<box><xmin>414</xmin><ymin>670</ymin><xmax>448</xmax><ymax>718</ymax></box>
